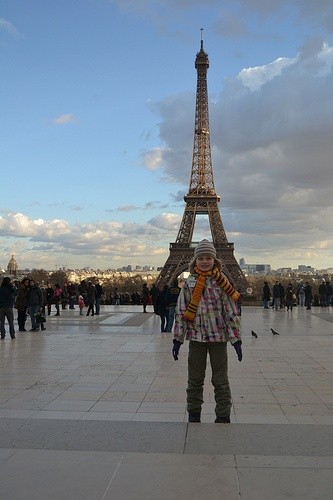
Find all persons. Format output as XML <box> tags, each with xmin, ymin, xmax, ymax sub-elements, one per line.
<box><xmin>0</xmin><ymin>277</ymin><xmax>140</xmax><ymax>341</ymax></box>
<box><xmin>262</xmin><ymin>281</ymin><xmax>271</xmax><ymax>309</ymax></box>
<box><xmin>172</xmin><ymin>239</ymin><xmax>243</xmax><ymax>424</ymax></box>
<box><xmin>271</xmin><ymin>281</ymin><xmax>333</xmax><ymax>312</ymax></box>
<box><xmin>141</xmin><ymin>283</ymin><xmax>150</xmax><ymax>313</ymax></box>
<box><xmin>149</xmin><ymin>279</ymin><xmax>181</xmax><ymax>333</ymax></box>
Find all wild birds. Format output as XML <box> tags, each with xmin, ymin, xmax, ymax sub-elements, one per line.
<box><xmin>251</xmin><ymin>330</ymin><xmax>257</xmax><ymax>338</ymax></box>
<box><xmin>270</xmin><ymin>328</ymin><xmax>280</xmax><ymax>336</ymax></box>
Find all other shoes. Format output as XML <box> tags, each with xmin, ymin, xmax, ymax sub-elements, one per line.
<box><xmin>69</xmin><ymin>307</ymin><xmax>75</xmax><ymax>309</ymax></box>
<box><xmin>19</xmin><ymin>329</ymin><xmax>27</xmax><ymax>331</ymax></box>
<box><xmin>306</xmin><ymin>307</ymin><xmax>311</xmax><ymax>310</ymax></box>
<box><xmin>1</xmin><ymin>337</ymin><xmax>4</xmax><ymax>339</ymax></box>
<box><xmin>12</xmin><ymin>336</ymin><xmax>15</xmax><ymax>339</ymax></box>
<box><xmin>287</xmin><ymin>308</ymin><xmax>289</xmax><ymax>311</ymax></box>
<box><xmin>54</xmin><ymin>313</ymin><xmax>60</xmax><ymax>316</ymax></box>
<box><xmin>29</xmin><ymin>328</ymin><xmax>36</xmax><ymax>332</ymax></box>
<box><xmin>91</xmin><ymin>314</ymin><xmax>94</xmax><ymax>316</ymax></box>
<box><xmin>42</xmin><ymin>328</ymin><xmax>46</xmax><ymax>331</ymax></box>
<box><xmin>94</xmin><ymin>313</ymin><xmax>98</xmax><ymax>315</ymax></box>
<box><xmin>62</xmin><ymin>308</ymin><xmax>66</xmax><ymax>309</ymax></box>
<box><xmin>291</xmin><ymin>308</ymin><xmax>292</xmax><ymax>311</ymax></box>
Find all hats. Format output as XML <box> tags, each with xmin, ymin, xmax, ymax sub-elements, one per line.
<box><xmin>79</xmin><ymin>295</ymin><xmax>83</xmax><ymax>298</ymax></box>
<box><xmin>194</xmin><ymin>239</ymin><xmax>216</xmax><ymax>259</ymax></box>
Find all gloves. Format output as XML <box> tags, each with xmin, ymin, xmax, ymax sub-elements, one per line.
<box><xmin>232</xmin><ymin>340</ymin><xmax>242</xmax><ymax>362</ymax></box>
<box><xmin>172</xmin><ymin>339</ymin><xmax>183</xmax><ymax>361</ymax></box>
